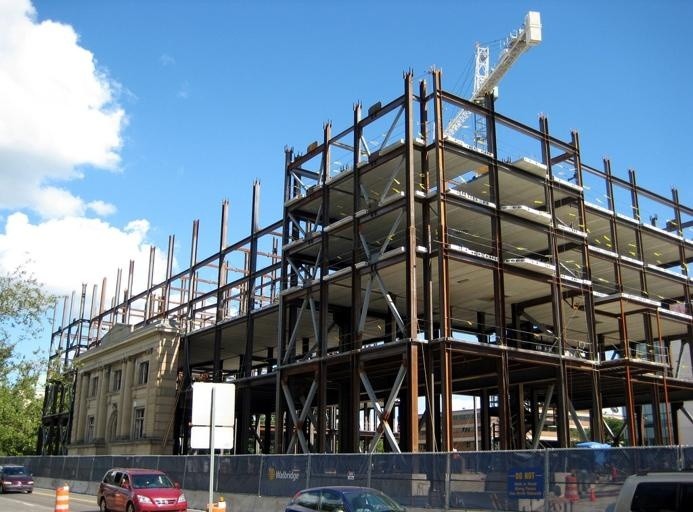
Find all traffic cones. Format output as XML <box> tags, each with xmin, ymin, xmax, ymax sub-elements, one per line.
<box><xmin>589</xmin><ymin>487</ymin><xmax>597</xmax><ymax>503</ymax></box>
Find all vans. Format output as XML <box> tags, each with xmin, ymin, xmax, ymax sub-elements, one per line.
<box><xmin>96</xmin><ymin>467</ymin><xmax>188</xmax><ymax>512</ymax></box>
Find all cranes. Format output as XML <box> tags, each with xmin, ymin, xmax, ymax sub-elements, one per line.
<box><xmin>428</xmin><ymin>9</ymin><xmax>543</xmax><ymax>155</ymax></box>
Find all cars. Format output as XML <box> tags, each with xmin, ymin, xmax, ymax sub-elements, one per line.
<box><xmin>283</xmin><ymin>483</ymin><xmax>410</xmax><ymax>512</ymax></box>
<box><xmin>0</xmin><ymin>464</ymin><xmax>34</xmax><ymax>494</ymax></box>
